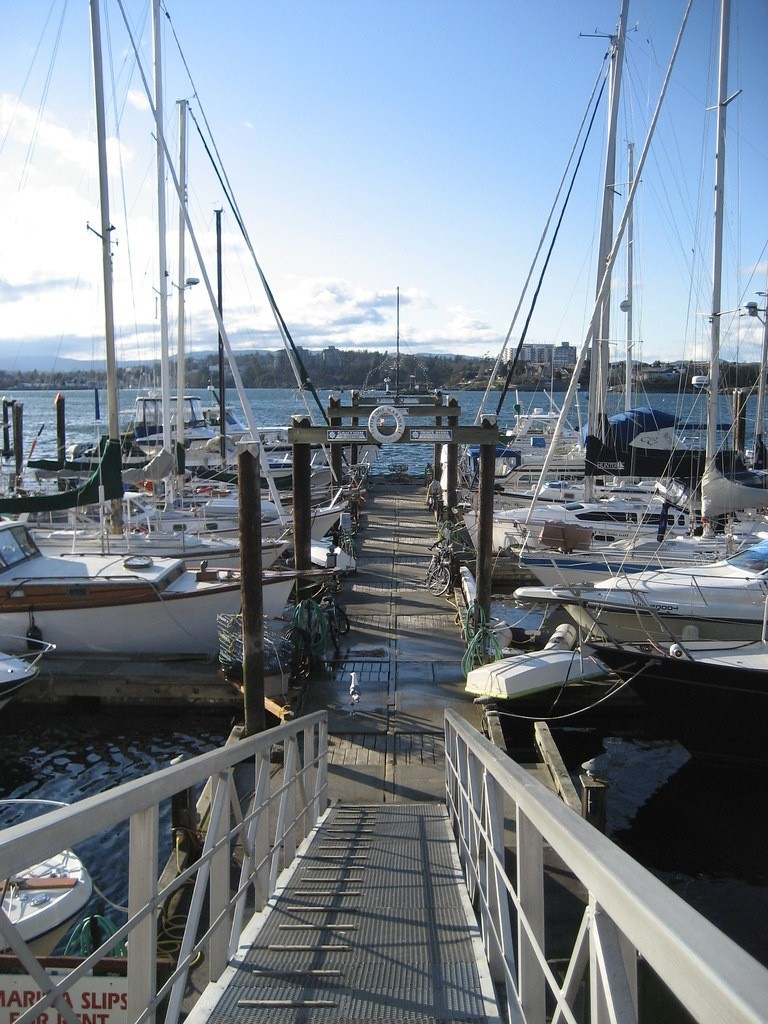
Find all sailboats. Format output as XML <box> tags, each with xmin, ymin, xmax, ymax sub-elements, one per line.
<box><xmin>454</xmin><ymin>1</ymin><xmax>766</xmax><ymax>737</ymax></box>
<box><xmin>0</xmin><ymin>0</ymin><xmax>347</xmax><ymax>709</ymax></box>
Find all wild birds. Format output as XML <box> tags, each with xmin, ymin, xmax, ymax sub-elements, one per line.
<box><xmin>350</xmin><ymin>671</ymin><xmax>361</xmax><ymax>706</ymax></box>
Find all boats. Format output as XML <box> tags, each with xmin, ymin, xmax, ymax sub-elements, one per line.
<box><xmin>0</xmin><ymin>848</ymin><xmax>93</xmax><ymax>958</ymax></box>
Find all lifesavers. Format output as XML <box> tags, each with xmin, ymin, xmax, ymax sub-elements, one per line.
<box><xmin>368</xmin><ymin>404</ymin><xmax>405</xmax><ymax>444</ymax></box>
